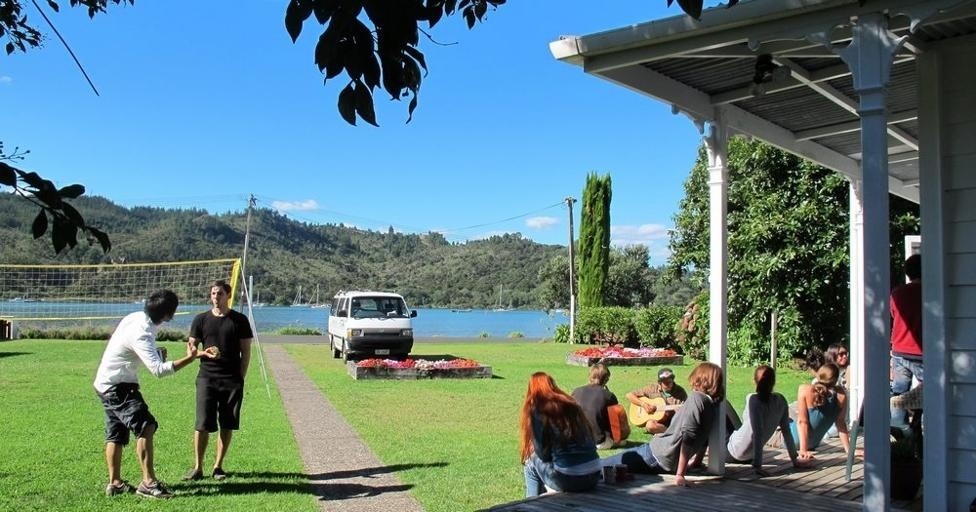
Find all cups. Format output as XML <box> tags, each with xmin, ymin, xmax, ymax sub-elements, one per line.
<box><xmin>156</xmin><ymin>347</ymin><xmax>166</xmax><ymax>363</ymax></box>
<box><xmin>603</xmin><ymin>466</ymin><xmax>613</xmax><ymax>481</ymax></box>
<box><xmin>615</xmin><ymin>465</ymin><xmax>627</xmax><ymax>480</ymax></box>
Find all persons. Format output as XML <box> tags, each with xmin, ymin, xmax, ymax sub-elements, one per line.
<box><xmin>519</xmin><ymin>370</ymin><xmax>601</xmax><ymax>499</ymax></box>
<box><xmin>625</xmin><ymin>368</ymin><xmax>688</xmax><ymax>434</ymax></box>
<box><xmin>571</xmin><ymin>362</ymin><xmax>629</xmax><ymax>450</ymax></box>
<box><xmin>725</xmin><ymin>365</ymin><xmax>814</xmax><ymax>478</ymax></box>
<box><xmin>765</xmin><ymin>253</ymin><xmax>924</xmax><ymax>460</ymax></box>
<box><xmin>92</xmin><ymin>289</ymin><xmax>197</xmax><ymax>501</ymax></box>
<box><xmin>599</xmin><ymin>362</ymin><xmax>743</xmax><ymax>489</ymax></box>
<box><xmin>181</xmin><ymin>279</ymin><xmax>253</xmax><ymax>481</ymax></box>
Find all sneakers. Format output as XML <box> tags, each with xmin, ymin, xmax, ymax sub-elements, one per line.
<box><xmin>105</xmin><ymin>481</ymin><xmax>137</xmax><ymax>499</ymax></box>
<box><xmin>135</xmin><ymin>481</ymin><xmax>173</xmax><ymax>500</ymax></box>
<box><xmin>211</xmin><ymin>468</ymin><xmax>225</xmax><ymax>480</ymax></box>
<box><xmin>181</xmin><ymin>470</ymin><xmax>202</xmax><ymax>481</ymax></box>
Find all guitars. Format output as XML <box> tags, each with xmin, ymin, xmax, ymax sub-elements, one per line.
<box><xmin>629</xmin><ymin>396</ymin><xmax>684</xmax><ymax>427</ymax></box>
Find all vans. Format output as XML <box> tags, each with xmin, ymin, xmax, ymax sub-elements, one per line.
<box><xmin>326</xmin><ymin>290</ymin><xmax>416</xmax><ymax>361</ymax></box>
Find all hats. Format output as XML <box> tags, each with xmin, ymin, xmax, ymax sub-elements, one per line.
<box><xmin>657</xmin><ymin>369</ymin><xmax>674</xmax><ymax>381</ymax></box>
